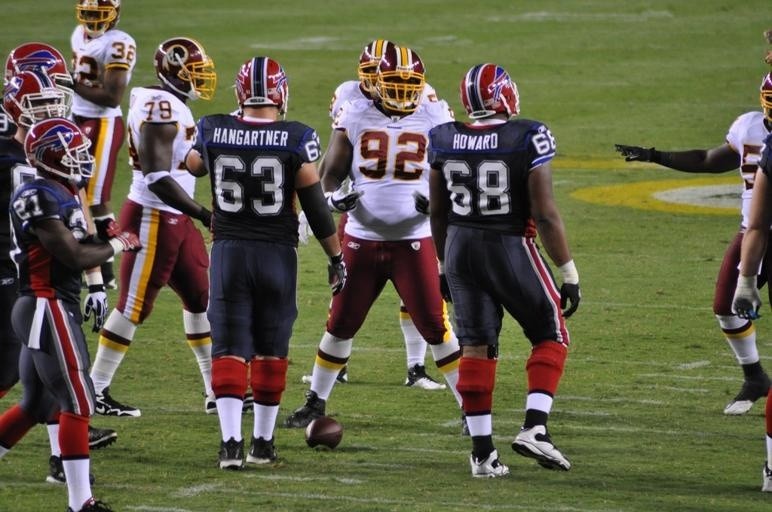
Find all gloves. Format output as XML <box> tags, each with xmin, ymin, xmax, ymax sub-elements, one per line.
<box><xmin>437</xmin><ymin>261</ymin><xmax>452</xmax><ymax>303</ymax></box>
<box><xmin>297</xmin><ymin>210</ymin><xmax>314</xmax><ymax>246</ymax></box>
<box><xmin>615</xmin><ymin>144</ymin><xmax>656</xmax><ymax>162</ymax></box>
<box><xmin>94</xmin><ymin>218</ymin><xmax>121</xmax><ymax>241</ymax></box>
<box><xmin>559</xmin><ymin>259</ymin><xmax>581</xmax><ymax>318</ymax></box>
<box><xmin>324</xmin><ymin>176</ymin><xmax>362</xmax><ymax>212</ymax></box>
<box><xmin>414</xmin><ymin>192</ymin><xmax>430</xmax><ymax>215</ymax></box>
<box><xmin>732</xmin><ymin>274</ymin><xmax>762</xmax><ymax>319</ymax></box>
<box><xmin>114</xmin><ymin>231</ymin><xmax>142</xmax><ymax>253</ymax></box>
<box><xmin>328</xmin><ymin>252</ymin><xmax>347</xmax><ymax>295</ymax></box>
<box><xmin>83</xmin><ymin>284</ymin><xmax>108</xmax><ymax>332</ymax></box>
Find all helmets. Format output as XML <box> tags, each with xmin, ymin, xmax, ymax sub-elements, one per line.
<box><xmin>358</xmin><ymin>40</ymin><xmax>426</xmax><ymax>116</ymax></box>
<box><xmin>0</xmin><ymin>42</ymin><xmax>96</xmax><ymax>192</ymax></box>
<box><xmin>155</xmin><ymin>37</ymin><xmax>217</xmax><ymax>101</ymax></box>
<box><xmin>76</xmin><ymin>0</ymin><xmax>120</xmax><ymax>38</ymax></box>
<box><xmin>236</xmin><ymin>56</ymin><xmax>288</xmax><ymax>120</ymax></box>
<box><xmin>759</xmin><ymin>72</ymin><xmax>772</xmax><ymax>128</ymax></box>
<box><xmin>460</xmin><ymin>63</ymin><xmax>521</xmax><ymax>119</ymax></box>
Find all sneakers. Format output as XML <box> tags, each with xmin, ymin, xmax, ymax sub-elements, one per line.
<box><xmin>470</xmin><ymin>449</ymin><xmax>509</xmax><ymax>478</ymax></box>
<box><xmin>206</xmin><ymin>365</ymin><xmax>349</xmax><ymax>470</ymax></box>
<box><xmin>461</xmin><ymin>411</ymin><xmax>470</xmax><ymax>434</ymax></box>
<box><xmin>723</xmin><ymin>373</ymin><xmax>771</xmax><ymax>415</ymax></box>
<box><xmin>46</xmin><ymin>387</ymin><xmax>141</xmax><ymax>512</ymax></box>
<box><xmin>762</xmin><ymin>461</ymin><xmax>771</xmax><ymax>491</ymax></box>
<box><xmin>83</xmin><ymin>278</ymin><xmax>117</xmax><ymax>291</ymax></box>
<box><xmin>405</xmin><ymin>363</ymin><xmax>447</xmax><ymax>390</ymax></box>
<box><xmin>512</xmin><ymin>424</ymin><xmax>571</xmax><ymax>471</ymax></box>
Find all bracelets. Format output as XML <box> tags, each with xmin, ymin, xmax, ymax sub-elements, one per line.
<box><xmin>201</xmin><ymin>206</ymin><xmax>212</xmax><ymax>227</ymax></box>
<box><xmin>558</xmin><ymin>259</ymin><xmax>579</xmax><ymax>285</ymax></box>
<box><xmin>436</xmin><ymin>256</ymin><xmax>445</xmax><ymax>275</ymax></box>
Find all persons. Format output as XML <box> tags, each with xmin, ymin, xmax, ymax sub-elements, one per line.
<box><xmin>615</xmin><ymin>71</ymin><xmax>772</xmax><ymax>415</ymax></box>
<box><xmin>89</xmin><ymin>38</ymin><xmax>254</xmax><ymax>418</ymax></box>
<box><xmin>731</xmin><ymin>134</ymin><xmax>772</xmax><ymax>492</ymax></box>
<box><xmin>427</xmin><ymin>63</ymin><xmax>582</xmax><ymax>479</ymax></box>
<box><xmin>186</xmin><ymin>57</ymin><xmax>347</xmax><ymax>469</ymax></box>
<box><xmin>284</xmin><ymin>46</ymin><xmax>469</xmax><ymax>435</ymax></box>
<box><xmin>0</xmin><ymin>0</ymin><xmax>142</xmax><ymax>512</ymax></box>
<box><xmin>301</xmin><ymin>39</ymin><xmax>447</xmax><ymax>391</ymax></box>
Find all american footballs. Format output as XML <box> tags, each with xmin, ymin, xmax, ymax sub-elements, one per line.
<box><xmin>306</xmin><ymin>416</ymin><xmax>341</xmax><ymax>449</ymax></box>
